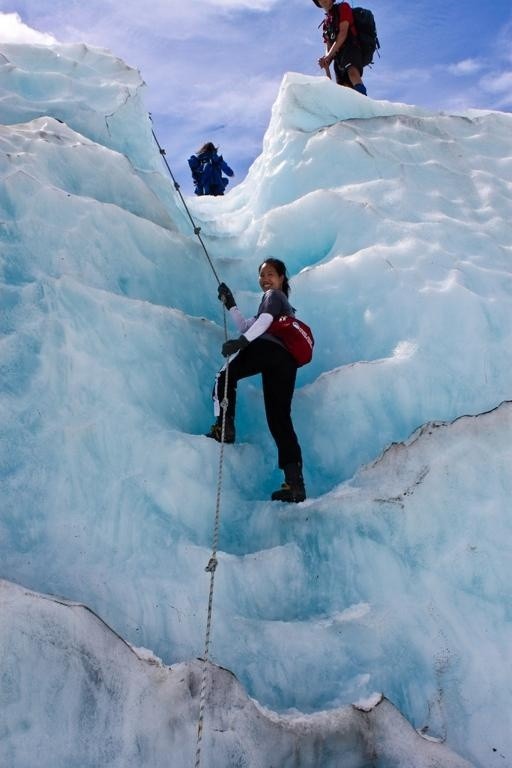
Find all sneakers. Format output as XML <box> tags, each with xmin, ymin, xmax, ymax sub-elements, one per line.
<box><xmin>203</xmin><ymin>423</ymin><xmax>236</xmax><ymax>444</ymax></box>
<box><xmin>271</xmin><ymin>486</ymin><xmax>307</xmax><ymax>504</ymax></box>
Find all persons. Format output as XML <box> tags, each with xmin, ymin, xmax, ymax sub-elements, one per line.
<box><xmin>206</xmin><ymin>257</ymin><xmax>316</xmax><ymax>503</ymax></box>
<box><xmin>197</xmin><ymin>143</ymin><xmax>234</xmax><ymax>195</ymax></box>
<box><xmin>312</xmin><ymin>0</ymin><xmax>366</xmax><ymax>97</ymax></box>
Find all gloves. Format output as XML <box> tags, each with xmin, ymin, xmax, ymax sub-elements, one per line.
<box><xmin>217</xmin><ymin>282</ymin><xmax>237</xmax><ymax>311</ymax></box>
<box><xmin>221</xmin><ymin>334</ymin><xmax>249</xmax><ymax>358</ymax></box>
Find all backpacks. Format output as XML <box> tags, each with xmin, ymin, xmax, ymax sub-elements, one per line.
<box><xmin>323</xmin><ymin>2</ymin><xmax>377</xmax><ymax>68</ymax></box>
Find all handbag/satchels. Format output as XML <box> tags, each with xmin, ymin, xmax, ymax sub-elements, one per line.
<box><xmin>269</xmin><ymin>316</ymin><xmax>315</xmax><ymax>367</ymax></box>
